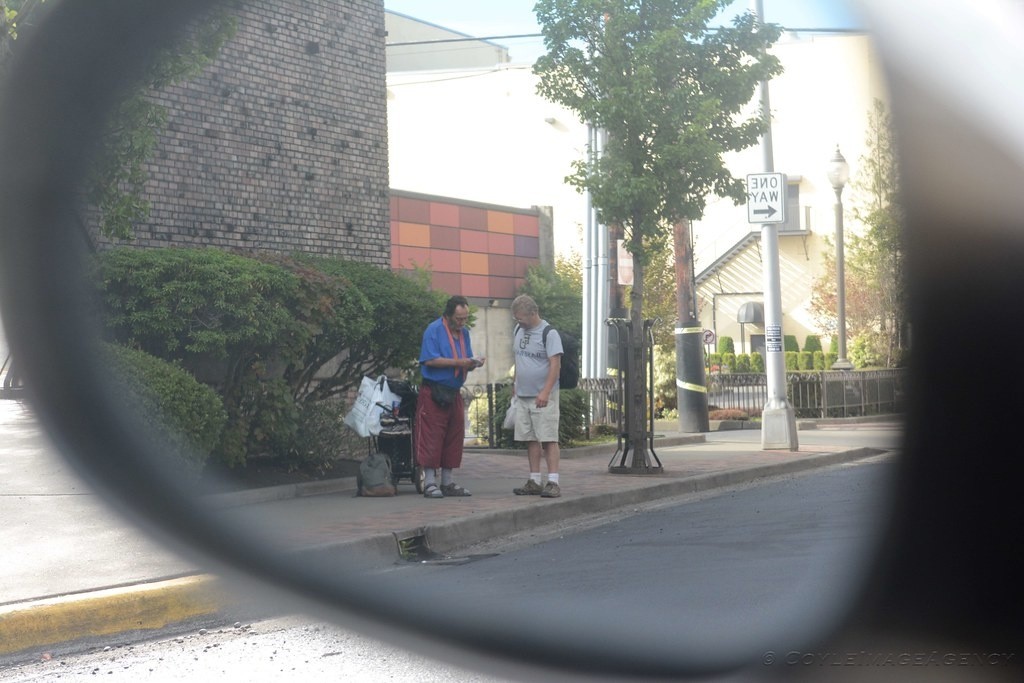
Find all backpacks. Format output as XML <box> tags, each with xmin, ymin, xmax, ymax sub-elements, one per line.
<box><xmin>515</xmin><ymin>325</ymin><xmax>580</xmax><ymax>389</ymax></box>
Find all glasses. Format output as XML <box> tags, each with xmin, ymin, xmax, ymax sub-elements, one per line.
<box><xmin>512</xmin><ymin>316</ymin><xmax>522</xmax><ymax>323</ymax></box>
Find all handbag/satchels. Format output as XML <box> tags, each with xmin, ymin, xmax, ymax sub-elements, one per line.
<box><xmin>359</xmin><ymin>436</ymin><xmax>396</xmax><ymax>497</ymax></box>
<box><xmin>502</xmin><ymin>395</ymin><xmax>518</xmax><ymax>431</ymax></box>
<box><xmin>425</xmin><ymin>381</ymin><xmax>457</xmax><ymax>408</ymax></box>
<box><xmin>344</xmin><ymin>375</ymin><xmax>417</xmax><ymax>438</ymax></box>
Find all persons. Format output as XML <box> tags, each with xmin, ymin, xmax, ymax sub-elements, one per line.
<box><xmin>510</xmin><ymin>295</ymin><xmax>564</xmax><ymax>497</ymax></box>
<box><xmin>413</xmin><ymin>296</ymin><xmax>485</xmax><ymax>498</ymax></box>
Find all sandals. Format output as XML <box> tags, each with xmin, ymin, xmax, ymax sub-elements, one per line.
<box><xmin>439</xmin><ymin>482</ymin><xmax>472</xmax><ymax>496</ymax></box>
<box><xmin>423</xmin><ymin>483</ymin><xmax>444</xmax><ymax>498</ymax></box>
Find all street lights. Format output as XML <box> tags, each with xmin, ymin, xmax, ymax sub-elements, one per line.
<box><xmin>830</xmin><ymin>144</ymin><xmax>854</xmax><ymax>370</ymax></box>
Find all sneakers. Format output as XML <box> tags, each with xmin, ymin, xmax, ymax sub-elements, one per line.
<box><xmin>513</xmin><ymin>479</ymin><xmax>543</xmax><ymax>495</ymax></box>
<box><xmin>540</xmin><ymin>481</ymin><xmax>561</xmax><ymax>497</ymax></box>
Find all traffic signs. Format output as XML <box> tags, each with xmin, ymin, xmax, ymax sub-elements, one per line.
<box><xmin>746</xmin><ymin>172</ymin><xmax>788</xmax><ymax>225</ymax></box>
